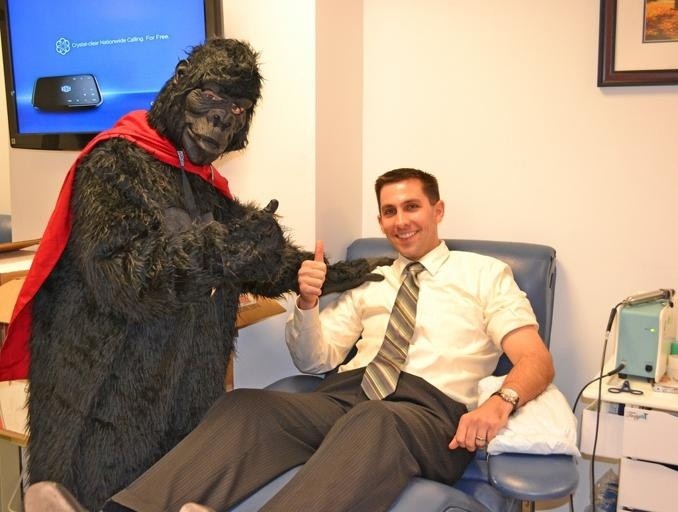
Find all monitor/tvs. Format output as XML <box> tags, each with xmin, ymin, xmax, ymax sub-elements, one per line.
<box><xmin>0</xmin><ymin>0</ymin><xmax>224</xmax><ymax>152</ymax></box>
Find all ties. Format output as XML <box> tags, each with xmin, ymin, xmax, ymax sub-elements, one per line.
<box><xmin>361</xmin><ymin>261</ymin><xmax>426</xmax><ymax>401</ymax></box>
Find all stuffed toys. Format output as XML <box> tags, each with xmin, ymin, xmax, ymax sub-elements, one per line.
<box><xmin>1</xmin><ymin>34</ymin><xmax>395</xmax><ymax>512</ymax></box>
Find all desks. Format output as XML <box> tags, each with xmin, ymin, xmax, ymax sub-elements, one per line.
<box><xmin>581</xmin><ymin>376</ymin><xmax>678</xmax><ymax>511</ymax></box>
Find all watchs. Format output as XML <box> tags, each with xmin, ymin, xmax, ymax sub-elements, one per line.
<box><xmin>490</xmin><ymin>387</ymin><xmax>519</xmax><ymax>416</ymax></box>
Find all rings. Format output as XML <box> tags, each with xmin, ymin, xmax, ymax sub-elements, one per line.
<box><xmin>475</xmin><ymin>435</ymin><xmax>487</xmax><ymax>441</ymax></box>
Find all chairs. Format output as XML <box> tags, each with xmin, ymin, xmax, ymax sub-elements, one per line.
<box><xmin>226</xmin><ymin>236</ymin><xmax>578</xmax><ymax>510</ymax></box>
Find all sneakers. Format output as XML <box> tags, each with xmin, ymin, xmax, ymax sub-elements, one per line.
<box><xmin>24</xmin><ymin>479</ymin><xmax>85</xmax><ymax>512</ymax></box>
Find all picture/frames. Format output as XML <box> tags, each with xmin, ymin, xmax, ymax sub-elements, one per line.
<box><xmin>598</xmin><ymin>1</ymin><xmax>677</xmax><ymax>88</ymax></box>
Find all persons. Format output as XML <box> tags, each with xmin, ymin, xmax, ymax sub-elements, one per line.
<box><xmin>23</xmin><ymin>164</ymin><xmax>555</xmax><ymax>512</ymax></box>
